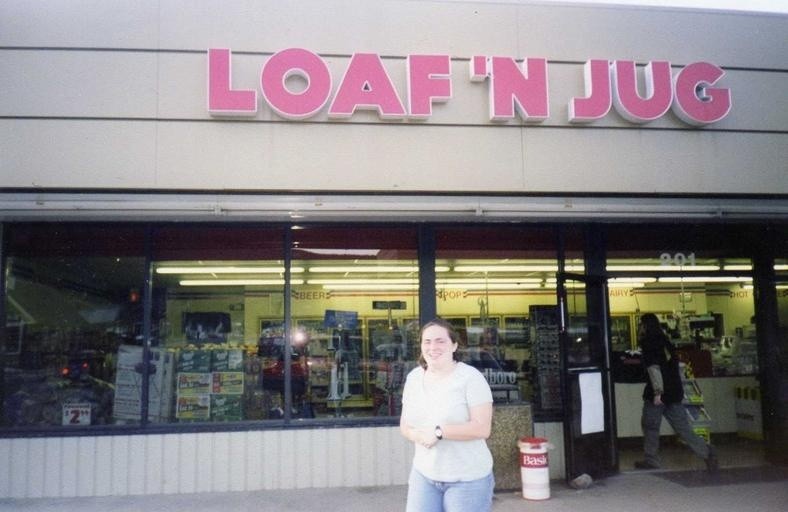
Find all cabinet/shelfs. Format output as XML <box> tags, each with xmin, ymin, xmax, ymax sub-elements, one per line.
<box><xmin>610</xmin><ymin>311</ymin><xmax>693</xmax><ymax>356</ymax></box>
<box><xmin>257</xmin><ymin>311</ymin><xmax>533</xmax><ymax>408</ymax></box>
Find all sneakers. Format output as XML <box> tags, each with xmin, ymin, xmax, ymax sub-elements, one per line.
<box><xmin>704</xmin><ymin>445</ymin><xmax>720</xmax><ymax>477</ymax></box>
<box><xmin>634</xmin><ymin>460</ymin><xmax>658</xmax><ymax>469</ymax></box>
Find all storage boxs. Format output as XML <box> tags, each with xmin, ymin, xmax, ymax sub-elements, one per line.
<box><xmin>174</xmin><ymin>348</ymin><xmax>248</xmax><ymax>422</ymax></box>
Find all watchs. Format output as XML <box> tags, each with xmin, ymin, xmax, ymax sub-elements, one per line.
<box><xmin>435</xmin><ymin>424</ymin><xmax>443</xmax><ymax>441</ymax></box>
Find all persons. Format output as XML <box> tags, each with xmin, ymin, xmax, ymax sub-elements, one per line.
<box><xmin>634</xmin><ymin>312</ymin><xmax>719</xmax><ymax>470</ymax></box>
<box><xmin>398</xmin><ymin>316</ymin><xmax>497</xmax><ymax>512</ymax></box>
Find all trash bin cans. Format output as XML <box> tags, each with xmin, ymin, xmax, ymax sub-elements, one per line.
<box><xmin>516</xmin><ymin>437</ymin><xmax>551</xmax><ymax>500</ymax></box>
